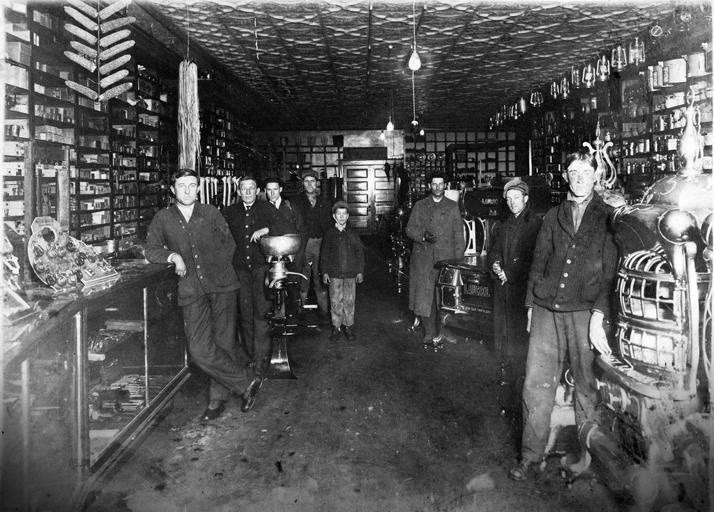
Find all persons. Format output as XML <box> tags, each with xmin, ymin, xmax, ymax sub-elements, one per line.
<box><xmin>507</xmin><ymin>153</ymin><xmax>618</xmax><ymax>481</ymax></box>
<box><xmin>263</xmin><ymin>177</ymin><xmax>307</xmax><ymax>275</ymax></box>
<box><xmin>144</xmin><ymin>169</ymin><xmax>263</xmax><ymax>414</ymax></box>
<box><xmin>487</xmin><ymin>179</ymin><xmax>544</xmax><ymax>439</ymax></box>
<box><xmin>289</xmin><ymin>170</ymin><xmax>331</xmax><ymax>325</ymax></box>
<box><xmin>405</xmin><ymin>171</ymin><xmax>466</xmax><ymax>353</ymax></box>
<box><xmin>320</xmin><ymin>201</ymin><xmax>365</xmax><ymax>342</ymax></box>
<box><xmin>220</xmin><ymin>174</ymin><xmax>299</xmax><ymax>382</ymax></box>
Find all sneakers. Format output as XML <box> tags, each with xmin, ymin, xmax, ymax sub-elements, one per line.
<box><xmin>344</xmin><ymin>327</ymin><xmax>356</xmax><ymax>340</ymax></box>
<box><xmin>331</xmin><ymin>329</ymin><xmax>342</xmax><ymax>342</ymax></box>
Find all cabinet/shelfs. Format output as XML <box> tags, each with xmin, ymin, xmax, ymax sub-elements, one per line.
<box><xmin>139</xmin><ymin>58</ymin><xmax>161</xmax><ymax>243</ymax></box>
<box><xmin>78</xmin><ymin>22</ymin><xmax>113</xmax><ymax>244</ymax></box>
<box><xmin>33</xmin><ymin>1</ymin><xmax>78</xmax><ymax>253</ymax></box>
<box><xmin>85</xmin><ymin>260</ymin><xmax>195</xmax><ymax>499</ymax></box>
<box><xmin>1</xmin><ymin>3</ymin><xmax>34</xmax><ymax>286</ymax></box>
<box><xmin>651</xmin><ymin>34</ymin><xmax>712</xmax><ymax>185</ymax></box>
<box><xmin>620</xmin><ymin>54</ymin><xmax>653</xmax><ymax>195</ymax></box>
<box><xmin>0</xmin><ymin>298</ymin><xmax>85</xmax><ymax>511</ymax></box>
<box><xmin>113</xmin><ymin>45</ymin><xmax>139</xmax><ymax>249</ymax></box>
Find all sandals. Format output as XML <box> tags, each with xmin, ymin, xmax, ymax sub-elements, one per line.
<box><xmin>511</xmin><ymin>460</ymin><xmax>539</xmax><ymax>481</ymax></box>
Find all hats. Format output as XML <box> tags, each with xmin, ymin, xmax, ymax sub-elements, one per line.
<box><xmin>615</xmin><ymin>215</ymin><xmax>656</xmax><ymax>259</ymax></box>
<box><xmin>504</xmin><ymin>177</ymin><xmax>528</xmax><ymax>199</ymax></box>
<box><xmin>303</xmin><ymin>170</ymin><xmax>319</xmax><ymax>180</ymax></box>
<box><xmin>333</xmin><ymin>201</ymin><xmax>349</xmax><ymax>214</ymax></box>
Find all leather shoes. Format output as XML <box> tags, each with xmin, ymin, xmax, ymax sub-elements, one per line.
<box><xmin>203</xmin><ymin>404</ymin><xmax>224</xmax><ymax>420</ymax></box>
<box><xmin>241</xmin><ymin>376</ymin><xmax>263</xmax><ymax>412</ymax></box>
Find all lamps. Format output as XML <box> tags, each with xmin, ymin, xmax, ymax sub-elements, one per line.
<box><xmin>533</xmin><ymin>91</ymin><xmax>543</xmax><ymax>107</ymax></box>
<box><xmin>495</xmin><ymin>112</ymin><xmax>502</xmax><ymax>126</ymax></box>
<box><xmin>560</xmin><ymin>78</ymin><xmax>570</xmax><ymax>100</ymax></box>
<box><xmin>489</xmin><ymin>117</ymin><xmax>493</xmax><ymax>130</ymax></box>
<box><xmin>582</xmin><ymin>65</ymin><xmax>595</xmax><ymax>88</ymax></box>
<box><xmin>597</xmin><ymin>55</ymin><xmax>610</xmax><ymax>82</ymax></box>
<box><xmin>386</xmin><ymin>114</ymin><xmax>393</xmax><ymax>131</ymax></box>
<box><xmin>409</xmin><ymin>3</ymin><xmax>421</xmax><ymax>71</ymax></box>
<box><xmin>501</xmin><ymin>105</ymin><xmax>508</xmax><ymax>119</ymax></box>
<box><xmin>412</xmin><ymin>71</ymin><xmax>419</xmax><ymax>126</ymax></box>
<box><xmin>513</xmin><ymin>103</ymin><xmax>519</xmax><ymax>119</ymax></box>
<box><xmin>411</xmin><ymin>69</ymin><xmax>418</xmax><ymax>126</ymax></box>
<box><xmin>551</xmin><ymin>82</ymin><xmax>559</xmax><ymax>98</ymax></box>
<box><xmin>612</xmin><ymin>46</ymin><xmax>627</xmax><ymax>72</ymax></box>
<box><xmin>572</xmin><ymin>68</ymin><xmax>582</xmax><ymax>88</ymax></box>
<box><xmin>530</xmin><ymin>92</ymin><xmax>538</xmax><ymax>106</ymax></box>
<box><xmin>629</xmin><ymin>37</ymin><xmax>645</xmax><ymax>66</ymax></box>
<box><xmin>509</xmin><ymin>105</ymin><xmax>514</xmax><ymax>116</ymax></box>
<box><xmin>520</xmin><ymin>96</ymin><xmax>526</xmax><ymax>114</ymax></box>
